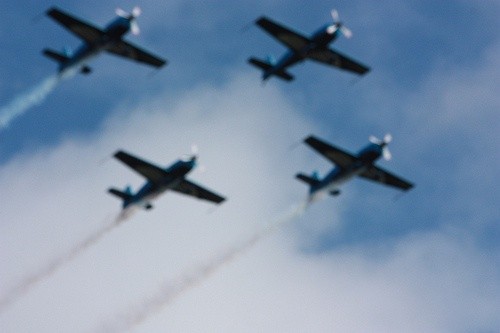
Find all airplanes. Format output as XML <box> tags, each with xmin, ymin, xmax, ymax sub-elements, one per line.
<box><xmin>295</xmin><ymin>134</ymin><xmax>415</xmax><ymax>204</ymax></box>
<box><xmin>40</xmin><ymin>6</ymin><xmax>169</xmax><ymax>79</ymax></box>
<box><xmin>247</xmin><ymin>8</ymin><xmax>371</xmax><ymax>83</ymax></box>
<box><xmin>107</xmin><ymin>151</ymin><xmax>225</xmax><ymax>211</ymax></box>
